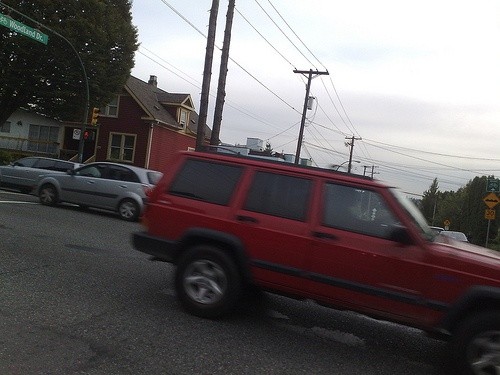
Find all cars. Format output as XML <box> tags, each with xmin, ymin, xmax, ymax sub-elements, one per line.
<box><xmin>439</xmin><ymin>230</ymin><xmax>471</xmax><ymax>243</ymax></box>
<box><xmin>32</xmin><ymin>162</ymin><xmax>163</xmax><ymax>222</ymax></box>
<box><xmin>0</xmin><ymin>156</ymin><xmax>84</xmax><ymax>194</ymax></box>
<box><xmin>429</xmin><ymin>226</ymin><xmax>445</xmax><ymax>236</ymax></box>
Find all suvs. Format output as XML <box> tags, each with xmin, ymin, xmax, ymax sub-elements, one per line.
<box><xmin>129</xmin><ymin>146</ymin><xmax>500</xmax><ymax>375</ymax></box>
